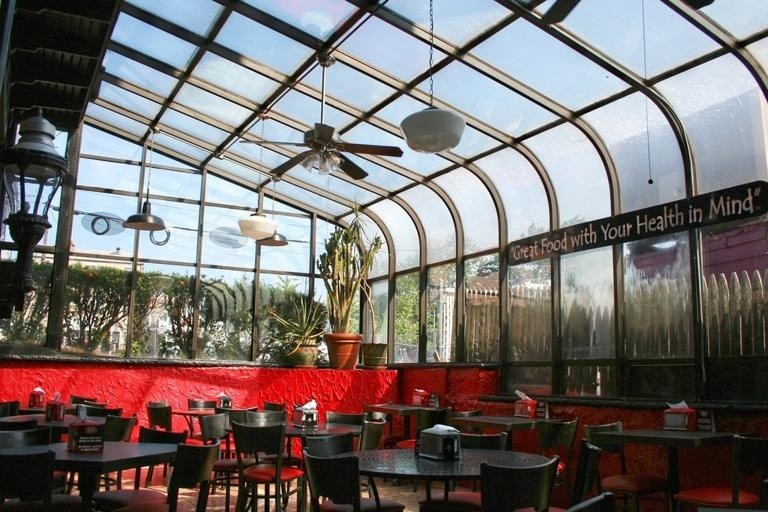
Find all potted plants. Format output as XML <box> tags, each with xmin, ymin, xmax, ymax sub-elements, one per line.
<box><xmin>358</xmin><ymin>274</ymin><xmax>390</xmax><ymax>369</ymax></box>
<box><xmin>316</xmin><ymin>216</ymin><xmax>382</xmax><ymax>369</ymax></box>
<box><xmin>269</xmin><ymin>298</ymin><xmax>326</xmax><ymax>365</ymax></box>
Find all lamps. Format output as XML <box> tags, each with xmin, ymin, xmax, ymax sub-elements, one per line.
<box><xmin>301</xmin><ymin>151</ymin><xmax>342</xmax><ymax>175</ymax></box>
<box><xmin>401</xmin><ymin>1</ymin><xmax>463</xmax><ymax>154</ymax></box>
<box><xmin>123</xmin><ymin>127</ymin><xmax>166</xmax><ymax>232</ymax></box>
<box><xmin>239</xmin><ymin>113</ymin><xmax>278</xmax><ymax>239</ymax></box>
<box><xmin>256</xmin><ymin>177</ymin><xmax>288</xmax><ymax>247</ymax></box>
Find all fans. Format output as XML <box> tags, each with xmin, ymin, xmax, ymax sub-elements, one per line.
<box><xmin>242</xmin><ymin>52</ymin><xmax>402</xmax><ymax>181</ymax></box>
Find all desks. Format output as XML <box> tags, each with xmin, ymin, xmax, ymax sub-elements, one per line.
<box><xmin>600</xmin><ymin>428</ymin><xmax>734</xmax><ymax>512</ymax></box>
<box><xmin>336</xmin><ymin>447</ymin><xmax>550</xmax><ymax>512</ymax></box>
<box><xmin>20</xmin><ymin>403</ymin><xmax>83</xmax><ymax>417</ymax></box>
<box><xmin>24</xmin><ymin>440</ymin><xmax>178</xmax><ymax>511</ymax></box>
<box><xmin>0</xmin><ymin>414</ymin><xmax>107</xmax><ymax>443</ymax></box>
<box><xmin>452</xmin><ymin>417</ymin><xmax>564</xmax><ymax>453</ymax></box>
<box><xmin>368</xmin><ymin>403</ymin><xmax>451</xmax><ymax>440</ymax></box>
<box><xmin>224</xmin><ymin>423</ymin><xmax>362</xmax><ymax>512</ymax></box>
<box><xmin>172</xmin><ymin>411</ymin><xmax>215</xmax><ymax>440</ymax></box>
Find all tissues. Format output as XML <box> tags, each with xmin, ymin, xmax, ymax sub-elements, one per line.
<box><xmin>217</xmin><ymin>391</ymin><xmax>232</xmax><ymax>407</ymax></box>
<box><xmin>412</xmin><ymin>389</ymin><xmax>429</xmax><ymax>406</ymax></box>
<box><xmin>66</xmin><ymin>405</ymin><xmax>104</xmax><ymax>453</ymax></box>
<box><xmin>663</xmin><ymin>400</ymin><xmax>696</xmax><ymax>431</ymax></box>
<box><xmin>45</xmin><ymin>390</ymin><xmax>65</xmax><ymax>422</ymax></box>
<box><xmin>292</xmin><ymin>397</ymin><xmax>319</xmax><ymax>427</ymax></box>
<box><xmin>28</xmin><ymin>386</ymin><xmax>45</xmax><ymax>409</ymax></box>
<box><xmin>418</xmin><ymin>423</ymin><xmax>462</xmax><ymax>460</ymax></box>
<box><xmin>513</xmin><ymin>389</ymin><xmax>537</xmax><ymax>417</ymax></box>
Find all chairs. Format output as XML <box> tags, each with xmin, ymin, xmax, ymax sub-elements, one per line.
<box><xmin>326</xmin><ymin>411</ymin><xmax>367</xmax><ymax>451</ymax></box>
<box><xmin>69</xmin><ymin>395</ymin><xmax>96</xmax><ymax>404</ymax></box>
<box><xmin>189</xmin><ymin>399</ymin><xmax>215</xmax><ymax>431</ymax></box>
<box><xmin>0</xmin><ymin>451</ymin><xmax>56</xmax><ymax>512</ymax></box>
<box><xmin>67</xmin><ymin>414</ymin><xmax>136</xmax><ymax>494</ymax></box>
<box><xmin>94</xmin><ymin>437</ymin><xmax>221</xmax><ymax>512</ymax></box>
<box><xmin>672</xmin><ymin>434</ymin><xmax>768</xmax><ymax>512</ymax></box>
<box><xmin>200</xmin><ymin>413</ymin><xmax>260</xmax><ymax>512</ymax></box>
<box><xmin>146</xmin><ymin>399</ymin><xmax>188</xmax><ymax>488</ymax></box>
<box><xmin>303</xmin><ymin>451</ymin><xmax>405</xmax><ymax>512</ymax></box>
<box><xmin>420</xmin><ymin>432</ymin><xmax>509</xmax><ymax>511</ymax></box>
<box><xmin>564</xmin><ymin>491</ymin><xmax>617</xmax><ymax>512</ymax></box>
<box><xmin>0</xmin><ymin>400</ymin><xmax>21</xmax><ymax>416</ymax></box>
<box><xmin>395</xmin><ymin>407</ymin><xmax>452</xmax><ymax>492</ymax></box>
<box><xmin>304</xmin><ymin>432</ymin><xmax>376</xmax><ymax>502</ymax></box>
<box><xmin>448</xmin><ymin>412</ymin><xmax>483</xmax><ymax>436</ymax></box>
<box><xmin>78</xmin><ymin>406</ymin><xmax>123</xmax><ymax>418</ymax></box>
<box><xmin>215</xmin><ymin>406</ymin><xmax>259</xmax><ymax>458</ymax></box>
<box><xmin>479</xmin><ymin>455</ymin><xmax>559</xmax><ymax>512</ymax></box>
<box><xmin>518</xmin><ymin>438</ymin><xmax>600</xmax><ymax>512</ymax></box>
<box><xmin>245</xmin><ymin>411</ymin><xmax>287</xmax><ymax>463</ymax></box>
<box><xmin>83</xmin><ymin>400</ymin><xmax>109</xmax><ymax>411</ymax></box>
<box><xmin>231</xmin><ymin>421</ymin><xmax>303</xmax><ymax>512</ymax></box>
<box><xmin>535</xmin><ymin>417</ymin><xmax>578</xmax><ymax>488</ymax></box>
<box><xmin>587</xmin><ymin>422</ymin><xmax>673</xmax><ymax>512</ymax></box>
<box><xmin>1</xmin><ymin>420</ymin><xmax>40</xmax><ymax>431</ymax></box>
<box><xmin>266</xmin><ymin>403</ymin><xmax>288</xmax><ymax>413</ymax></box>
